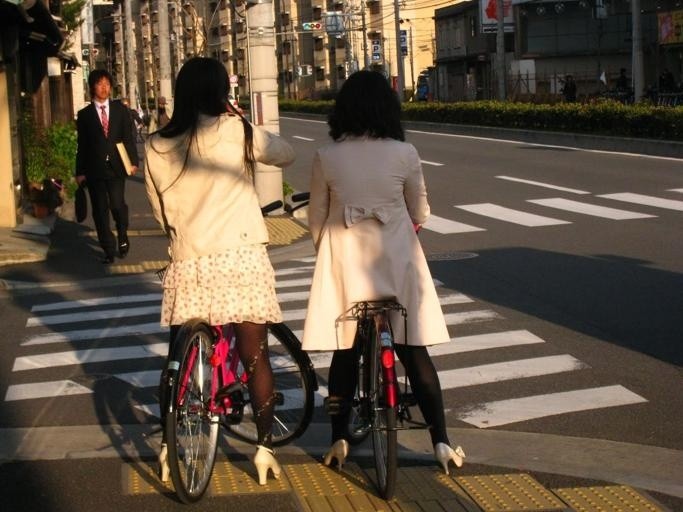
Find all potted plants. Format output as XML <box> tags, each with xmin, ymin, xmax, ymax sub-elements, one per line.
<box><xmin>17</xmin><ymin>113</ymin><xmax>78</xmax><ymax>220</ymax></box>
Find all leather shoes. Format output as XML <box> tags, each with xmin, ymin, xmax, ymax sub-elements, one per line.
<box><xmin>101</xmin><ymin>235</ymin><xmax>130</xmax><ymax>264</ymax></box>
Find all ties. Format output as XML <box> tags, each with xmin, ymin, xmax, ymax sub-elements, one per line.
<box><xmin>100</xmin><ymin>105</ymin><xmax>109</xmax><ymax>140</ymax></box>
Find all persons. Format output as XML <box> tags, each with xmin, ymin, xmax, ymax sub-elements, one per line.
<box><xmin>661</xmin><ymin>68</ymin><xmax>676</xmax><ymax>90</ymax></box>
<box><xmin>417</xmin><ymin>81</ymin><xmax>429</xmax><ymax>102</ymax></box>
<box><xmin>121</xmin><ymin>96</ymin><xmax>173</xmax><ymax>135</ymax></box>
<box><xmin>301</xmin><ymin>71</ymin><xmax>465</xmax><ymax>475</ymax></box>
<box><xmin>614</xmin><ymin>68</ymin><xmax>629</xmax><ymax>87</ymax></box>
<box><xmin>143</xmin><ymin>58</ymin><xmax>296</xmax><ymax>485</ymax></box>
<box><xmin>75</xmin><ymin>69</ymin><xmax>138</xmax><ymax>263</ymax></box>
<box><xmin>561</xmin><ymin>75</ymin><xmax>577</xmax><ymax>102</ymax></box>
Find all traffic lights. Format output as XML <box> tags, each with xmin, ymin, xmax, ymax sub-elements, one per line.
<box><xmin>302</xmin><ymin>22</ymin><xmax>323</xmax><ymax>30</ymax></box>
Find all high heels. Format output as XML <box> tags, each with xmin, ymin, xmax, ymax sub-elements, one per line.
<box><xmin>157</xmin><ymin>442</ymin><xmax>171</xmax><ymax>485</ymax></box>
<box><xmin>434</xmin><ymin>442</ymin><xmax>467</xmax><ymax>476</ymax></box>
<box><xmin>254</xmin><ymin>445</ymin><xmax>282</xmax><ymax>486</ymax></box>
<box><xmin>322</xmin><ymin>438</ymin><xmax>349</xmax><ymax>470</ymax></box>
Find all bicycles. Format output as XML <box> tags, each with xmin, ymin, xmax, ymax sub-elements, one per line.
<box><xmin>159</xmin><ymin>199</ymin><xmax>318</xmax><ymax>505</ymax></box>
<box><xmin>293</xmin><ymin>192</ymin><xmax>423</xmax><ymax>500</ymax></box>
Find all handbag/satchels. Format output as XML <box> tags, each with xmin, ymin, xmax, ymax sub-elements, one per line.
<box><xmin>75</xmin><ymin>186</ymin><xmax>87</xmax><ymax>223</ymax></box>
